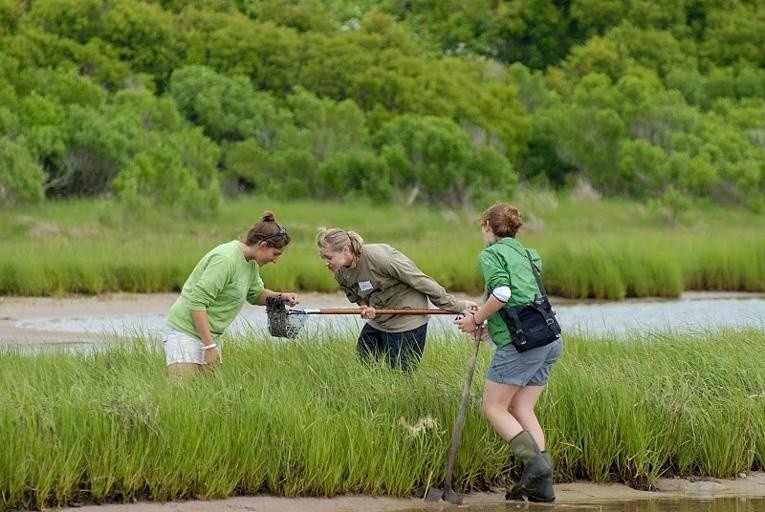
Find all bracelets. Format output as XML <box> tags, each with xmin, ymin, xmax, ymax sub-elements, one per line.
<box><xmin>277</xmin><ymin>292</ymin><xmax>283</xmax><ymax>300</ymax></box>
<box><xmin>470</xmin><ymin>315</ymin><xmax>480</xmax><ymax>327</ymax></box>
<box><xmin>202</xmin><ymin>342</ymin><xmax>217</xmax><ymax>351</ymax></box>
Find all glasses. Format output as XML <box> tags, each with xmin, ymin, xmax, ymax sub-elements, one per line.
<box><xmin>258</xmin><ymin>226</ymin><xmax>287</xmax><ymax>247</ymax></box>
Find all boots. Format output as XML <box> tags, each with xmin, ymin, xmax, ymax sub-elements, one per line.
<box><xmin>504</xmin><ymin>451</ymin><xmax>556</xmax><ymax>502</ymax></box>
<box><xmin>506</xmin><ymin>429</ymin><xmax>551</xmax><ymax>495</ymax></box>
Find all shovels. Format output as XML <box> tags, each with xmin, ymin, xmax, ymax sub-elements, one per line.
<box><xmin>265</xmin><ymin>296</ymin><xmax>461</xmax><ymax>338</ymax></box>
<box><xmin>423</xmin><ymin>320</ymin><xmax>483</xmax><ymax>505</ymax></box>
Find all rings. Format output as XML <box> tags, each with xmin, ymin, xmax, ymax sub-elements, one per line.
<box><xmin>365</xmin><ymin>312</ymin><xmax>369</xmax><ymax>316</ymax></box>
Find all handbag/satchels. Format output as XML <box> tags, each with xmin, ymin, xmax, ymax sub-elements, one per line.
<box><xmin>502</xmin><ymin>294</ymin><xmax>562</xmax><ymax>353</ymax></box>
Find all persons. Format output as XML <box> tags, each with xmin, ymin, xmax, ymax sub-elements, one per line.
<box><xmin>161</xmin><ymin>210</ymin><xmax>292</xmax><ymax>381</ymax></box>
<box><xmin>312</xmin><ymin>224</ymin><xmax>477</xmax><ymax>374</ymax></box>
<box><xmin>452</xmin><ymin>201</ymin><xmax>564</xmax><ymax>502</ymax></box>
